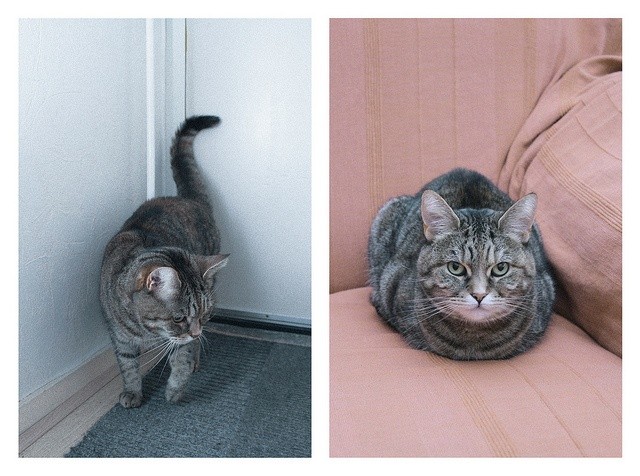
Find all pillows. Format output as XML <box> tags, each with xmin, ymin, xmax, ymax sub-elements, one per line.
<box><xmin>500</xmin><ymin>54</ymin><xmax>623</xmax><ymax>356</ymax></box>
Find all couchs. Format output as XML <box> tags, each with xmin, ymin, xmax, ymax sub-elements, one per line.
<box><xmin>329</xmin><ymin>19</ymin><xmax>623</xmax><ymax>456</ymax></box>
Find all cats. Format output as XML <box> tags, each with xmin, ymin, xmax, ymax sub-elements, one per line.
<box><xmin>368</xmin><ymin>168</ymin><xmax>555</xmax><ymax>359</ymax></box>
<box><xmin>100</xmin><ymin>115</ymin><xmax>231</xmax><ymax>407</ymax></box>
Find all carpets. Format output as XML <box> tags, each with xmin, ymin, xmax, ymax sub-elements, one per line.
<box><xmin>65</xmin><ymin>330</ymin><xmax>311</xmax><ymax>457</ymax></box>
